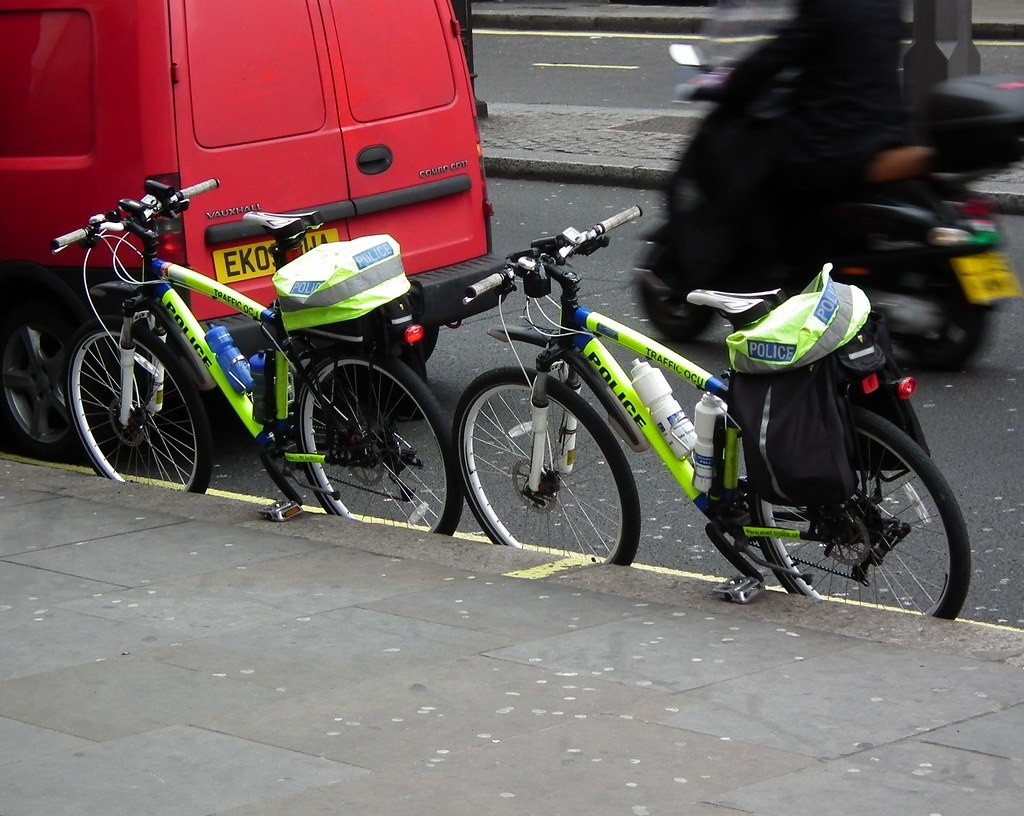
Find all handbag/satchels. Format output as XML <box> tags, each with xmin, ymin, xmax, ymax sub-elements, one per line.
<box><xmin>730</xmin><ymin>313</ymin><xmax>929</xmax><ymax>508</ymax></box>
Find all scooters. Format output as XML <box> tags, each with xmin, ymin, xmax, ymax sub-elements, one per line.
<box><xmin>636</xmin><ymin>42</ymin><xmax>1024</xmax><ymax>378</ymax></box>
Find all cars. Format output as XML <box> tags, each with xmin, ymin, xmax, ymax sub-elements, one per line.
<box><xmin>0</xmin><ymin>1</ymin><xmax>519</xmax><ymax>465</ymax></box>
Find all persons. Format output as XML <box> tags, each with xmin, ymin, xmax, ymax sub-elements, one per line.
<box><xmin>660</xmin><ymin>1</ymin><xmax>911</xmax><ymax>294</ymax></box>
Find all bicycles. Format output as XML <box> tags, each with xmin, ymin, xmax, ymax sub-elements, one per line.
<box><xmin>47</xmin><ymin>177</ymin><xmax>462</xmax><ymax>536</ymax></box>
<box><xmin>451</xmin><ymin>203</ymin><xmax>973</xmax><ymax>620</ymax></box>
